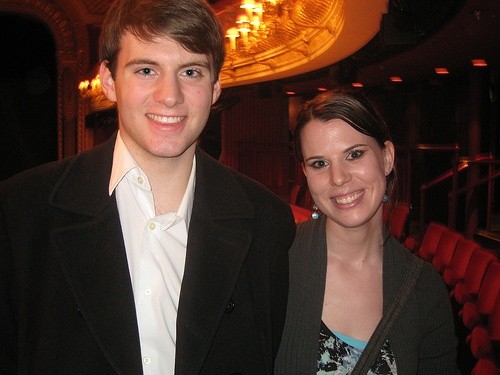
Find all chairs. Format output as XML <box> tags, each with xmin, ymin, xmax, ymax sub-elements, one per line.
<box><xmin>290</xmin><ymin>185</ymin><xmax>500</xmax><ymax>375</ymax></box>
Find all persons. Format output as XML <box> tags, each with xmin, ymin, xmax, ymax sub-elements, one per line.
<box><xmin>271</xmin><ymin>86</ymin><xmax>459</xmax><ymax>375</ymax></box>
<box><xmin>0</xmin><ymin>0</ymin><xmax>297</xmax><ymax>375</ymax></box>
